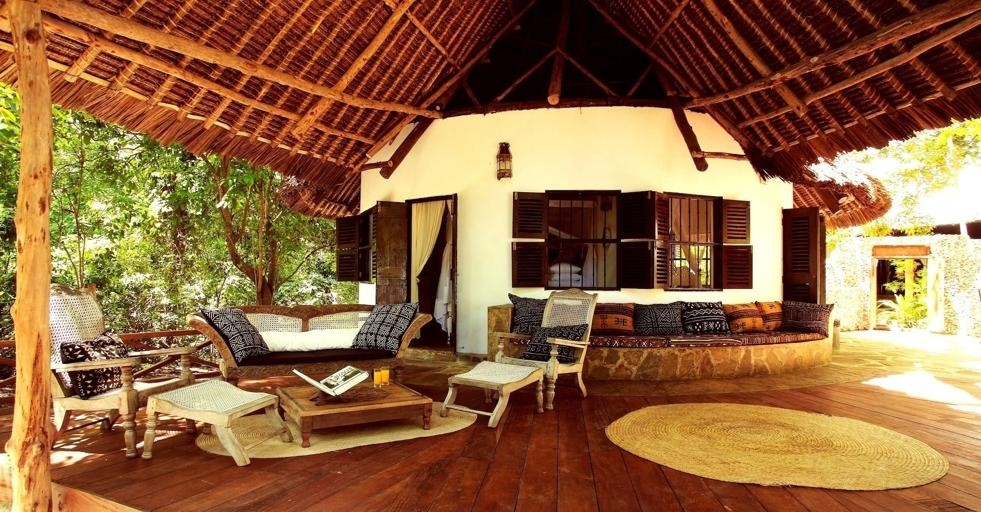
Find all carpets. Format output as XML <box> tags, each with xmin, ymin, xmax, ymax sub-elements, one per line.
<box><xmin>195</xmin><ymin>401</ymin><xmax>480</xmax><ymax>460</ymax></box>
<box><xmin>605</xmin><ymin>400</ymin><xmax>951</xmax><ymax>492</ymax></box>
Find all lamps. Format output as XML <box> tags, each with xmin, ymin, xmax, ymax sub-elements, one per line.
<box><xmin>495</xmin><ymin>141</ymin><xmax>513</xmax><ymax>181</ymax></box>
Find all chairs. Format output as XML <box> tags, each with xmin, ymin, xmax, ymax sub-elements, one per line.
<box><xmin>45</xmin><ymin>282</ymin><xmax>198</xmax><ymax>460</ymax></box>
<box><xmin>491</xmin><ymin>287</ymin><xmax>601</xmax><ymax>409</ymax></box>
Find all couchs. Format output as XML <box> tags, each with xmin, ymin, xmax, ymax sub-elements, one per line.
<box><xmin>183</xmin><ymin>301</ymin><xmax>434</xmax><ymax>385</ymax></box>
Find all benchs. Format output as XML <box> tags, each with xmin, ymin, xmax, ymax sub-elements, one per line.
<box><xmin>486</xmin><ymin>302</ymin><xmax>837</xmax><ymax>380</ymax></box>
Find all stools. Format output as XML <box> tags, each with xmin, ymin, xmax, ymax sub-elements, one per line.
<box><xmin>440</xmin><ymin>360</ymin><xmax>547</xmax><ymax>429</ymax></box>
<box><xmin>141</xmin><ymin>376</ymin><xmax>296</xmax><ymax>467</ymax></box>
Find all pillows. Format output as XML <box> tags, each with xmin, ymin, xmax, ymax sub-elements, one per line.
<box><xmin>782</xmin><ymin>298</ymin><xmax>836</xmax><ymax>338</ymax></box>
<box><xmin>350</xmin><ymin>301</ymin><xmax>421</xmax><ymax>358</ymax></box>
<box><xmin>551</xmin><ymin>262</ymin><xmax>581</xmax><ymax>273</ymax></box>
<box><xmin>754</xmin><ymin>300</ymin><xmax>785</xmax><ymax>332</ymax></box>
<box><xmin>201</xmin><ymin>307</ymin><xmax>272</xmax><ymax>363</ymax></box>
<box><xmin>680</xmin><ymin>300</ymin><xmax>733</xmax><ymax>335</ymax></box>
<box><xmin>507</xmin><ymin>291</ymin><xmax>553</xmax><ymax>334</ymax></box>
<box><xmin>552</xmin><ymin>273</ymin><xmax>581</xmax><ymax>282</ymax></box>
<box><xmin>590</xmin><ymin>301</ymin><xmax>637</xmax><ymax>336</ymax></box>
<box><xmin>518</xmin><ymin>324</ymin><xmax>589</xmax><ymax>363</ymax></box>
<box><xmin>59</xmin><ymin>327</ymin><xmax>135</xmax><ymax>400</ymax></box>
<box><xmin>722</xmin><ymin>302</ymin><xmax>766</xmax><ymax>333</ymax></box>
<box><xmin>634</xmin><ymin>300</ymin><xmax>687</xmax><ymax>336</ymax></box>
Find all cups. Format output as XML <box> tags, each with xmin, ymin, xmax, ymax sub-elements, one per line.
<box><xmin>381</xmin><ymin>365</ymin><xmax>391</xmax><ymax>385</ymax></box>
<box><xmin>374</xmin><ymin>368</ymin><xmax>381</xmax><ymax>390</ymax></box>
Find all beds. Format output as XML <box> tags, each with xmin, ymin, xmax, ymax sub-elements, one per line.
<box><xmin>547</xmin><ymin>281</ymin><xmax>581</xmax><ymax>288</ymax></box>
